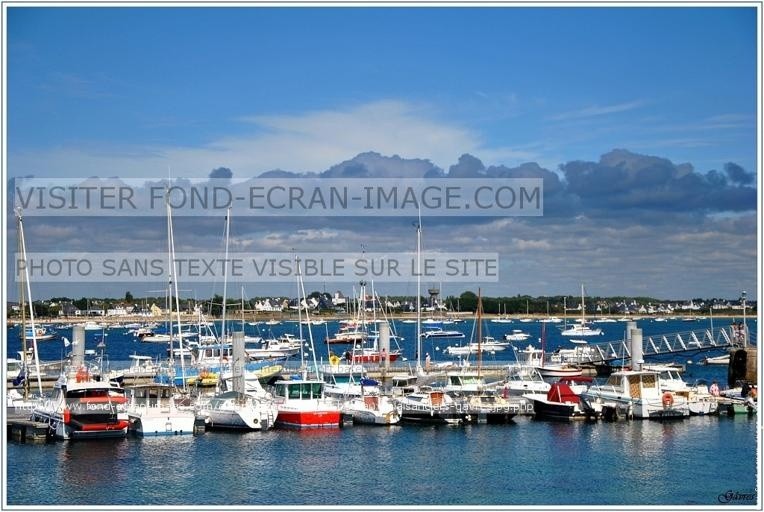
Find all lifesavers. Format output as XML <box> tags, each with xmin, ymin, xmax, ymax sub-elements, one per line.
<box><xmin>662</xmin><ymin>393</ymin><xmax>673</xmax><ymax>405</ymax></box>
<box><xmin>330</xmin><ymin>356</ymin><xmax>338</xmax><ymax>366</ymax></box>
<box><xmin>621</xmin><ymin>366</ymin><xmax>631</xmax><ymax>371</ymax></box>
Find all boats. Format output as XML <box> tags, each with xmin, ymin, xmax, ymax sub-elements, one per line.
<box><xmin>506</xmin><ymin>283</ymin><xmax>757</xmax><ymax>419</ymax></box>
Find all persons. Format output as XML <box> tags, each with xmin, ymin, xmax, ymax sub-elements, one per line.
<box><xmin>731</xmin><ymin>322</ymin><xmax>739</xmax><ymax>340</ymax></box>
<box><xmin>709</xmin><ymin>380</ymin><xmax>719</xmax><ymax>396</ymax></box>
<box><xmin>744</xmin><ymin>392</ymin><xmax>756</xmax><ymax>406</ymax></box>
<box><xmin>425</xmin><ymin>351</ymin><xmax>432</xmax><ymax>370</ymax></box>
<box><xmin>379</xmin><ymin>348</ymin><xmax>387</xmax><ymax>368</ymax></box>
<box><xmin>738</xmin><ymin>321</ymin><xmax>745</xmax><ymax>342</ymax></box>
